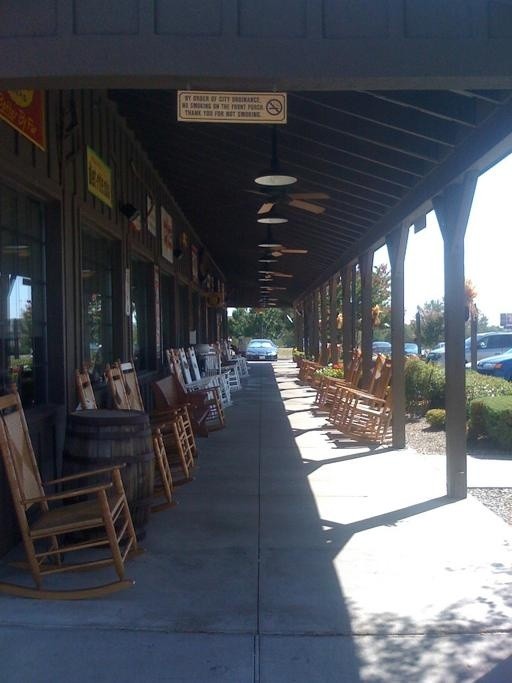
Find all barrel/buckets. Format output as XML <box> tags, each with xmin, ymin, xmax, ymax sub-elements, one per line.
<box><xmin>61</xmin><ymin>409</ymin><xmax>155</xmax><ymax>547</ymax></box>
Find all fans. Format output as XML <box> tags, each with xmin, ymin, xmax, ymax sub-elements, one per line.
<box><xmin>240</xmin><ymin>122</ymin><xmax>328</xmax><ymax>308</ymax></box>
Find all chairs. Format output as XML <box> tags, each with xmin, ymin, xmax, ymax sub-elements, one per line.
<box><xmin>1</xmin><ymin>391</ymin><xmax>148</xmax><ymax>600</ymax></box>
<box><xmin>294</xmin><ymin>342</ymin><xmax>393</xmax><ymax>442</ymax></box>
<box><xmin>75</xmin><ymin>339</ymin><xmax>249</xmax><ymax>514</ymax></box>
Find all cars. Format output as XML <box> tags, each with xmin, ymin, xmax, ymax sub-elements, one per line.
<box><xmin>404</xmin><ymin>341</ymin><xmax>418</xmax><ymax>354</ymax></box>
<box><xmin>419</xmin><ymin>328</ymin><xmax>511</xmax><ymax>384</ymax></box>
<box><xmin>244</xmin><ymin>339</ymin><xmax>279</xmax><ymax>360</ymax></box>
<box><xmin>372</xmin><ymin>340</ymin><xmax>392</xmax><ymax>354</ymax></box>
